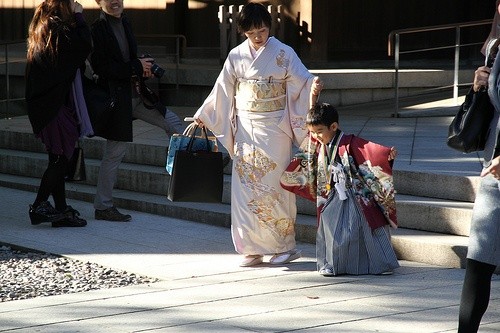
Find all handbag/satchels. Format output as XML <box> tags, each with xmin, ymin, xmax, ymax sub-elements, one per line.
<box><xmin>165</xmin><ymin>122</ymin><xmax>215</xmax><ymax>175</ymax></box>
<box><xmin>69</xmin><ymin>138</ymin><xmax>87</xmax><ymax>181</ymax></box>
<box><xmin>167</xmin><ymin>122</ymin><xmax>223</xmax><ymax>203</ymax></box>
<box><xmin>445</xmin><ymin>38</ymin><xmax>500</xmax><ymax>153</ymax></box>
<box><xmin>173</xmin><ymin>122</ymin><xmax>218</xmax><ymax>152</ymax></box>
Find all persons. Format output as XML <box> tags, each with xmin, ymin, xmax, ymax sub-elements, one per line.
<box><xmin>458</xmin><ymin>0</ymin><xmax>500</xmax><ymax>333</ymax></box>
<box><xmin>27</xmin><ymin>0</ymin><xmax>92</xmax><ymax>227</ymax></box>
<box><xmin>80</xmin><ymin>0</ymin><xmax>186</xmax><ymax>221</ymax></box>
<box><xmin>193</xmin><ymin>2</ymin><xmax>322</xmax><ymax>266</ymax></box>
<box><xmin>279</xmin><ymin>77</ymin><xmax>399</xmax><ymax>276</ymax></box>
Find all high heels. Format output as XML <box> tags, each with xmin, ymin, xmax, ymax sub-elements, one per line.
<box><xmin>28</xmin><ymin>200</ymin><xmax>68</xmax><ymax>225</ymax></box>
<box><xmin>52</xmin><ymin>205</ymin><xmax>86</xmax><ymax>227</ymax></box>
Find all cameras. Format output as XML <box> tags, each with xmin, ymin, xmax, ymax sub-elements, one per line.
<box><xmin>140</xmin><ymin>54</ymin><xmax>165</xmax><ymax>78</ymax></box>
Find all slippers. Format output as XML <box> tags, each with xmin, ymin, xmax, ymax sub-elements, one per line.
<box><xmin>319</xmin><ymin>265</ymin><xmax>335</xmax><ymax>277</ymax></box>
<box><xmin>238</xmin><ymin>255</ymin><xmax>263</xmax><ymax>266</ymax></box>
<box><xmin>270</xmin><ymin>249</ymin><xmax>302</xmax><ymax>265</ymax></box>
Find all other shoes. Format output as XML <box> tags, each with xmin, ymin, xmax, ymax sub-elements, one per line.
<box><xmin>95</xmin><ymin>206</ymin><xmax>132</xmax><ymax>221</ymax></box>
